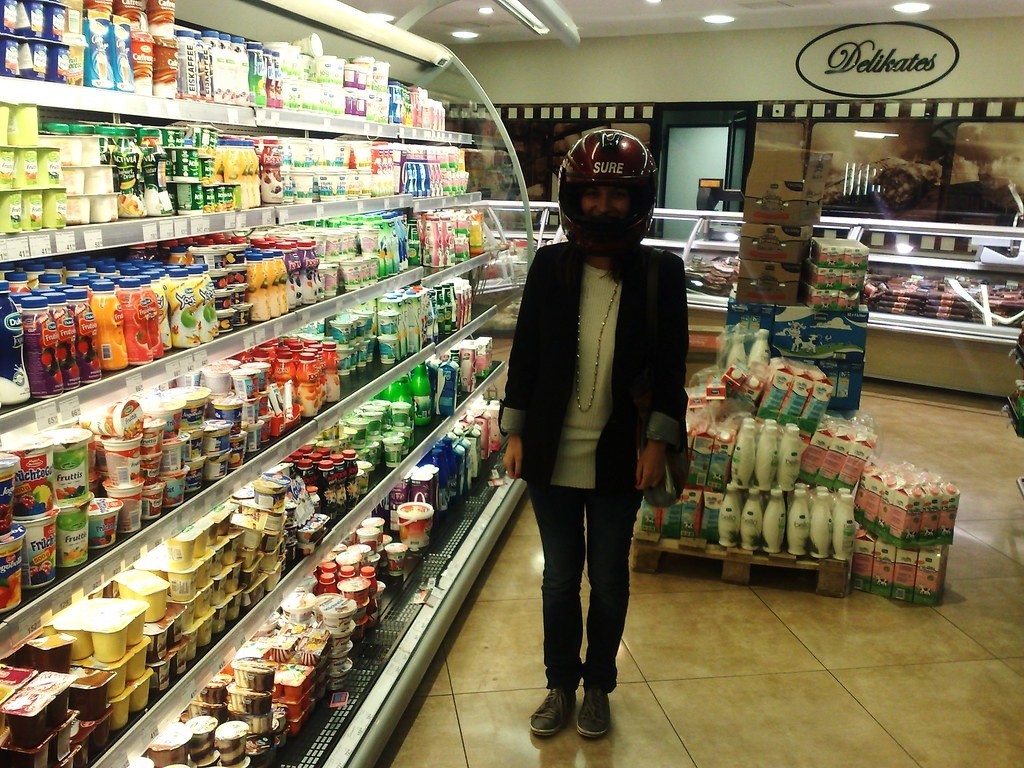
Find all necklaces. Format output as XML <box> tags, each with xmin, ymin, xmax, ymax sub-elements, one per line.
<box><xmin>576</xmin><ymin>284</ymin><xmax>618</xmax><ymax>412</ymax></box>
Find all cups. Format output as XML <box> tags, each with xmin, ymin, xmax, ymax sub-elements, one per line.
<box><xmin>306</xmin><ymin>400</ymin><xmax>412</xmax><ymax>494</ymax></box>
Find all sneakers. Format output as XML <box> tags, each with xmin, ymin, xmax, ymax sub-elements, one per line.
<box><xmin>528</xmin><ymin>685</ymin><xmax>577</xmax><ymax>737</ymax></box>
<box><xmin>575</xmin><ymin>687</ymin><xmax>610</xmax><ymax>739</ymax></box>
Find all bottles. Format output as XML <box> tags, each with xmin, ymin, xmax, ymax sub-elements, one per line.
<box><xmin>214</xmin><ymin>137</ymin><xmax>282</xmax><ymax>209</ymax></box>
<box><xmin>300</xmin><ymin>209</ymin><xmax>455</xmax><ymax>278</ymax></box>
<box><xmin>283</xmin><ymin>446</ymin><xmax>359</xmax><ymax>522</ymax></box>
<box><xmin>40</xmin><ymin>123</ymin><xmax>173</xmax><ymax>218</ymax></box>
<box><xmin>0</xmin><ymin>232</ymin><xmax>325</xmax><ymax>405</ymax></box>
<box><xmin>726</xmin><ymin>335</ymin><xmax>747</xmax><ymax>371</ymax></box>
<box><xmin>174</xmin><ymin>30</ymin><xmax>282</xmax><ymax>108</ymax></box>
<box><xmin>813</xmin><ymin>155</ymin><xmax>824</xmax><ymax>180</ymax></box>
<box><xmin>368</xmin><ymin>361</ymin><xmax>432</xmax><ymax>426</ymax></box>
<box><xmin>231</xmin><ymin>338</ymin><xmax>340</xmax><ymax>417</ymax></box>
<box><xmin>787</xmin><ymin>483</ymin><xmax>855</xmax><ymax>561</ymax></box>
<box><xmin>748</xmin><ymin>329</ymin><xmax>771</xmax><ymax>368</ymax></box>
<box><xmin>718</xmin><ymin>486</ymin><xmax>784</xmax><ymax>553</ymax></box>
<box><xmin>731</xmin><ymin>419</ymin><xmax>803</xmax><ymax>492</ymax></box>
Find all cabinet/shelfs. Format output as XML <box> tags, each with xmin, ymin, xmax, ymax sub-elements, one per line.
<box><xmin>0</xmin><ymin>0</ymin><xmax>579</xmax><ymax>768</ymax></box>
<box><xmin>1006</xmin><ymin>332</ymin><xmax>1024</xmax><ymax>499</ymax></box>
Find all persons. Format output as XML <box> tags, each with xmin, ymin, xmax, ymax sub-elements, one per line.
<box><xmin>498</xmin><ymin>128</ymin><xmax>689</xmax><ymax>738</ymax></box>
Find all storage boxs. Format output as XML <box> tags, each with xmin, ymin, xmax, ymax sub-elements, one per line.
<box><xmin>721</xmin><ymin>144</ymin><xmax>869</xmax><ymax>420</ymax></box>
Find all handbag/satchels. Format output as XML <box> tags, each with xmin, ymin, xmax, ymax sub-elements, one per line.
<box><xmin>629</xmin><ymin>386</ymin><xmax>692</xmax><ymax>506</ymax></box>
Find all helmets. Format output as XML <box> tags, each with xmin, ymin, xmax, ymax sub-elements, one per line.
<box><xmin>557</xmin><ymin>129</ymin><xmax>660</xmax><ymax>255</ymax></box>
